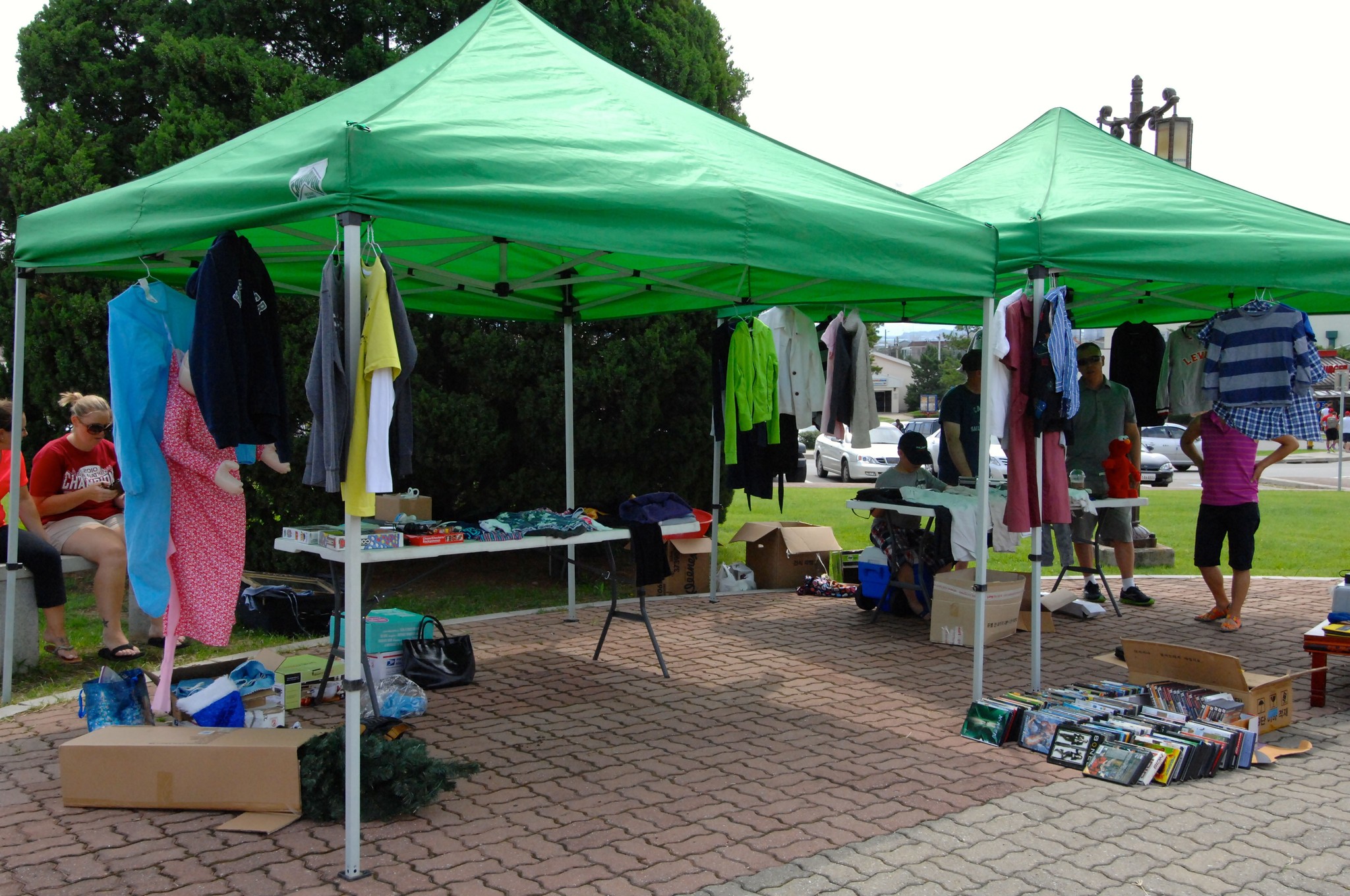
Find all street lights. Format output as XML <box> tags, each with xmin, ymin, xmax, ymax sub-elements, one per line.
<box><xmin>894</xmin><ymin>337</ymin><xmax>899</xmax><ymax>358</ymax></box>
<box><xmin>936</xmin><ymin>333</ymin><xmax>941</xmax><ymax>363</ymax></box>
<box><xmin>884</xmin><ymin>329</ymin><xmax>890</xmax><ymax>355</ymax></box>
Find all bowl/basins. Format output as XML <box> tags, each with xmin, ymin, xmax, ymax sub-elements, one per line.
<box><xmin>663</xmin><ymin>508</ymin><xmax>713</xmax><ymax>540</ymax></box>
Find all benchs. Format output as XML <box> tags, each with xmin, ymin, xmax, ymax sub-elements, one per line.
<box><xmin>1</xmin><ymin>554</ymin><xmax>150</xmax><ymax>675</ymax></box>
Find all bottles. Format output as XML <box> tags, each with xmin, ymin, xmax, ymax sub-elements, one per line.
<box><xmin>1331</xmin><ymin>570</ymin><xmax>1350</xmax><ymax>613</ymax></box>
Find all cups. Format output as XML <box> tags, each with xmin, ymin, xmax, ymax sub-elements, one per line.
<box><xmin>1069</xmin><ymin>470</ymin><xmax>1085</xmax><ymax>490</ymax></box>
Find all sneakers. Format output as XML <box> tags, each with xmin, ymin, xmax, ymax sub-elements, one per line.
<box><xmin>1083</xmin><ymin>580</ymin><xmax>1106</xmax><ymax>602</ymax></box>
<box><xmin>1119</xmin><ymin>584</ymin><xmax>1155</xmax><ymax>605</ymax></box>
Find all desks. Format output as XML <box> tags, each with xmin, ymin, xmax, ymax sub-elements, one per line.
<box><xmin>274</xmin><ymin>528</ymin><xmax>671</xmax><ymax>716</ymax></box>
<box><xmin>846</xmin><ymin>495</ymin><xmax>1150</xmax><ymax>625</ymax></box>
<box><xmin>1303</xmin><ymin>619</ymin><xmax>1350</xmax><ymax>709</ymax></box>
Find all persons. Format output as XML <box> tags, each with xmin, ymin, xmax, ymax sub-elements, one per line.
<box><xmin>1333</xmin><ymin>411</ymin><xmax>1345</xmax><ymax>449</ymax></box>
<box><xmin>150</xmin><ymin>342</ymin><xmax>290</xmax><ymax>715</ymax></box>
<box><xmin>1180</xmin><ymin>409</ymin><xmax>1299</xmax><ymax>631</ymax></box>
<box><xmin>1058</xmin><ymin>732</ymin><xmax>1088</xmax><ymax>745</ymax></box>
<box><xmin>29</xmin><ymin>392</ymin><xmax>190</xmax><ymax>661</ymax></box>
<box><xmin>1023</xmin><ymin>716</ymin><xmax>1057</xmax><ymax>750</ymax></box>
<box><xmin>1338</xmin><ymin>411</ymin><xmax>1350</xmax><ymax>453</ymax></box>
<box><xmin>1114</xmin><ymin>745</ymin><xmax>1138</xmax><ymax>753</ymax></box>
<box><xmin>1321</xmin><ymin>403</ymin><xmax>1330</xmax><ymax>433</ymax></box>
<box><xmin>871</xmin><ymin>432</ymin><xmax>955</xmax><ymax>620</ymax></box>
<box><xmin>1319</xmin><ymin>408</ymin><xmax>1338</xmax><ymax>453</ymax></box>
<box><xmin>1054</xmin><ymin>748</ymin><xmax>1082</xmax><ymax>760</ymax></box>
<box><xmin>896</xmin><ymin>419</ymin><xmax>903</xmax><ymax>431</ymax></box>
<box><xmin>0</xmin><ymin>399</ymin><xmax>82</xmax><ymax>664</ymax></box>
<box><xmin>1318</xmin><ymin>400</ymin><xmax>1333</xmax><ymax>416</ymax></box>
<box><xmin>1060</xmin><ymin>342</ymin><xmax>1155</xmax><ymax>606</ymax></box>
<box><xmin>937</xmin><ymin>349</ymin><xmax>1007</xmax><ymax>572</ymax></box>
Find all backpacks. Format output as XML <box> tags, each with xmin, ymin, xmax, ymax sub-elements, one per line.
<box><xmin>1327</xmin><ymin>415</ymin><xmax>1337</xmax><ymax>428</ymax></box>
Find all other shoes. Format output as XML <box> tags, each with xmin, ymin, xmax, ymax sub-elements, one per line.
<box><xmin>1220</xmin><ymin>615</ymin><xmax>1242</xmax><ymax>632</ymax></box>
<box><xmin>1327</xmin><ymin>451</ymin><xmax>1330</xmax><ymax>453</ymax></box>
<box><xmin>1331</xmin><ymin>447</ymin><xmax>1335</xmax><ymax>452</ymax></box>
<box><xmin>1194</xmin><ymin>602</ymin><xmax>1231</xmax><ymax>622</ymax></box>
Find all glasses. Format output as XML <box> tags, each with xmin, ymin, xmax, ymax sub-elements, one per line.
<box><xmin>75</xmin><ymin>415</ymin><xmax>114</xmax><ymax>435</ymax></box>
<box><xmin>1077</xmin><ymin>355</ymin><xmax>1102</xmax><ymax>365</ymax></box>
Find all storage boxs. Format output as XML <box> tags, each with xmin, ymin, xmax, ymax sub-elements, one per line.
<box><xmin>361</xmin><ymin>648</ymin><xmax>403</xmax><ymax>683</ymax></box>
<box><xmin>59</xmin><ymin>723</ymin><xmax>336</xmax><ymax>836</ymax></box>
<box><xmin>728</xmin><ymin>521</ymin><xmax>842</xmax><ymax>589</ymax></box>
<box><xmin>403</xmin><ymin>531</ymin><xmax>465</xmax><ymax>547</ymax></box>
<box><xmin>319</xmin><ymin>528</ymin><xmax>403</xmax><ymax>551</ymax></box>
<box><xmin>275</xmin><ymin>655</ymin><xmax>344</xmax><ymax>709</ymax></box>
<box><xmin>1040</xmin><ymin>592</ymin><xmax>1106</xmax><ymax>619</ymax></box>
<box><xmin>1093</xmin><ymin>637</ymin><xmax>1326</xmax><ymax>735</ymax></box>
<box><xmin>170</xmin><ymin>648</ymin><xmax>286</xmax><ymax>720</ymax></box>
<box><xmin>329</xmin><ymin>607</ymin><xmax>434</xmax><ymax>653</ymax></box>
<box><xmin>1008</xmin><ymin>572</ymin><xmax>1079</xmax><ymax>633</ymax></box>
<box><xmin>929</xmin><ymin>568</ymin><xmax>1026</xmax><ymax>646</ymax></box>
<box><xmin>622</xmin><ymin>535</ymin><xmax>724</xmax><ymax>596</ymax></box>
<box><xmin>375</xmin><ymin>493</ymin><xmax>432</xmax><ymax>521</ymax></box>
<box><xmin>242</xmin><ymin>700</ymin><xmax>287</xmax><ymax>727</ymax></box>
<box><xmin>282</xmin><ymin>524</ymin><xmax>343</xmax><ymax>544</ymax></box>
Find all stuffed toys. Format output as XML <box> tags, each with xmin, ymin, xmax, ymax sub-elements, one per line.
<box><xmin>1102</xmin><ymin>435</ymin><xmax>1141</xmax><ymax>498</ymax></box>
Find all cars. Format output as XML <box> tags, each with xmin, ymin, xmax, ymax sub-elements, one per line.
<box><xmin>901</xmin><ymin>418</ymin><xmax>1011</xmax><ymax>480</ymax></box>
<box><xmin>815</xmin><ymin>421</ymin><xmax>926</xmax><ymax>483</ymax></box>
<box><xmin>1140</xmin><ymin>443</ymin><xmax>1174</xmax><ymax>488</ymax></box>
<box><xmin>772</xmin><ymin>441</ymin><xmax>807</xmax><ymax>482</ymax></box>
<box><xmin>1140</xmin><ymin>422</ymin><xmax>1206</xmax><ymax>472</ymax></box>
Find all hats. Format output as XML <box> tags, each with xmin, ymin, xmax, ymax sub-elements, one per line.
<box><xmin>175</xmin><ymin>674</ymin><xmax>245</xmax><ymax>728</ymax></box>
<box><xmin>1328</xmin><ymin>408</ymin><xmax>1334</xmax><ymax>412</ymax></box>
<box><xmin>960</xmin><ymin>349</ymin><xmax>982</xmax><ymax>372</ymax></box>
<box><xmin>898</xmin><ymin>431</ymin><xmax>933</xmax><ymax>465</ymax></box>
<box><xmin>1345</xmin><ymin>411</ymin><xmax>1350</xmax><ymax>415</ymax></box>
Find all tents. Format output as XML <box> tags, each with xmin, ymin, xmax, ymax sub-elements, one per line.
<box><xmin>708</xmin><ymin>106</ymin><xmax>1350</xmax><ymax>694</ymax></box>
<box><xmin>0</xmin><ymin>0</ymin><xmax>1002</xmax><ymax>881</ymax></box>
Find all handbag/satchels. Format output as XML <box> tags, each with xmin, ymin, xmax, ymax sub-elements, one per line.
<box><xmin>170</xmin><ymin>660</ymin><xmax>275</xmax><ymax>697</ymax></box>
<box><xmin>401</xmin><ymin>616</ymin><xmax>475</xmax><ymax>689</ymax></box>
<box><xmin>79</xmin><ymin>668</ymin><xmax>155</xmax><ymax>732</ymax></box>
<box><xmin>240</xmin><ymin>589</ymin><xmax>322</xmax><ymax>639</ymax></box>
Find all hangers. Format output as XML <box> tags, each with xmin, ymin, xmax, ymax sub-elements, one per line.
<box><xmin>730</xmin><ymin>271</ymin><xmax>1282</xmax><ymax>335</ymax></box>
<box><xmin>134</xmin><ymin>212</ymin><xmax>386</xmax><ymax>288</ymax></box>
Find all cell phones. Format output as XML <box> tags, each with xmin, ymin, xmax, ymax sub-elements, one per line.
<box><xmin>108</xmin><ymin>480</ymin><xmax>125</xmax><ymax>496</ymax></box>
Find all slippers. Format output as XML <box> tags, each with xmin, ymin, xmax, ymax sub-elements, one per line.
<box><xmin>917</xmin><ymin>609</ymin><xmax>932</xmax><ymax>620</ymax></box>
<box><xmin>44</xmin><ymin>645</ymin><xmax>83</xmax><ymax>665</ymax></box>
<box><xmin>98</xmin><ymin>643</ymin><xmax>146</xmax><ymax>662</ymax></box>
<box><xmin>148</xmin><ymin>636</ymin><xmax>191</xmax><ymax>649</ymax></box>
